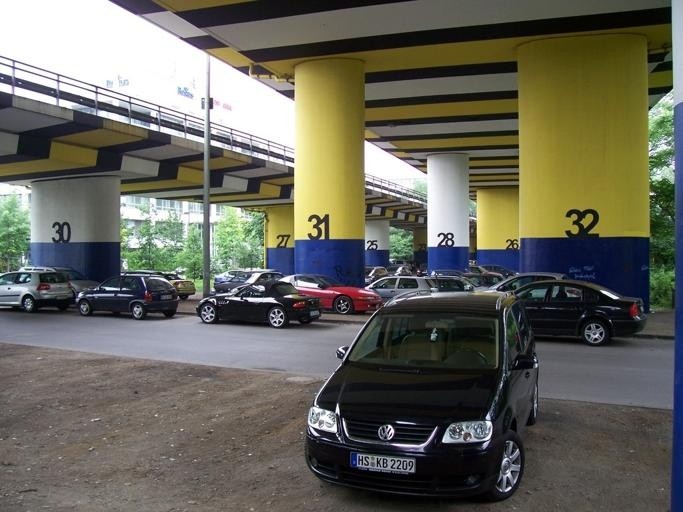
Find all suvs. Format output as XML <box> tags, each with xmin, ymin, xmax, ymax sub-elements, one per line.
<box><xmin>305</xmin><ymin>288</ymin><xmax>540</xmax><ymax>500</ymax></box>
<box><xmin>0</xmin><ymin>269</ymin><xmax>197</xmax><ymax>320</ymax></box>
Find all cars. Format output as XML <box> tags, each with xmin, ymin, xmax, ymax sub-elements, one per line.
<box><xmin>214</xmin><ymin>269</ymin><xmax>284</xmax><ymax>289</ymax></box>
<box><xmin>196</xmin><ymin>279</ymin><xmax>322</xmax><ymax>329</ymax></box>
<box><xmin>513</xmin><ymin>280</ymin><xmax>646</xmax><ymax>346</ymax></box>
<box><xmin>278</xmin><ymin>273</ymin><xmax>383</xmax><ymax>316</ymax></box>
<box><xmin>366</xmin><ymin>268</ymin><xmax>572</xmax><ymax>306</ymax></box>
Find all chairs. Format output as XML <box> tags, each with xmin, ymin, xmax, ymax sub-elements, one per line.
<box><xmin>396</xmin><ymin>332</ymin><xmax>496</xmax><ymax>368</ymax></box>
<box><xmin>554</xmin><ymin>292</ymin><xmax>567</xmax><ymax>301</ymax></box>
